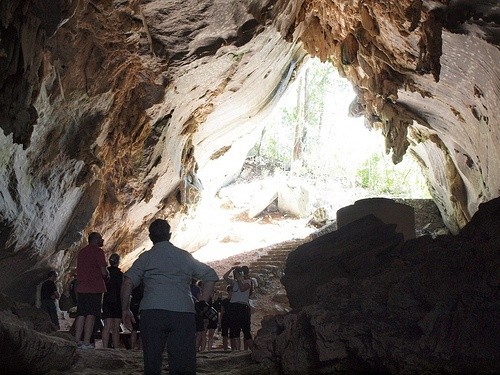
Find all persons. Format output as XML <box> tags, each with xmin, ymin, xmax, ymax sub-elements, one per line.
<box><xmin>241</xmin><ymin>265</ymin><xmax>256</xmax><ymax>351</ymax></box>
<box><xmin>41</xmin><ymin>271</ymin><xmax>60</xmax><ymax>330</ymax></box>
<box><xmin>223</xmin><ymin>266</ymin><xmax>251</xmax><ymax>352</ymax></box>
<box><xmin>191</xmin><ymin>280</ymin><xmax>233</xmax><ymax>357</ymax></box>
<box><xmin>102</xmin><ymin>254</ymin><xmax>123</xmax><ymax>348</ymax></box>
<box><xmin>70</xmin><ymin>232</ymin><xmax>110</xmax><ymax>349</ymax></box>
<box><xmin>121</xmin><ymin>219</ymin><xmax>218</xmax><ymax>375</ymax></box>
<box><xmin>130</xmin><ymin>283</ymin><xmax>143</xmax><ymax>350</ymax></box>
<box><xmin>221</xmin><ymin>286</ymin><xmax>234</xmax><ymax>350</ymax></box>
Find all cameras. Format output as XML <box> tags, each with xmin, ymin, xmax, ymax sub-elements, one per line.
<box><xmin>195</xmin><ymin>300</ymin><xmax>218</xmax><ymax>320</ymax></box>
<box><xmin>236</xmin><ymin>268</ymin><xmax>244</xmax><ymax>276</ymax></box>
